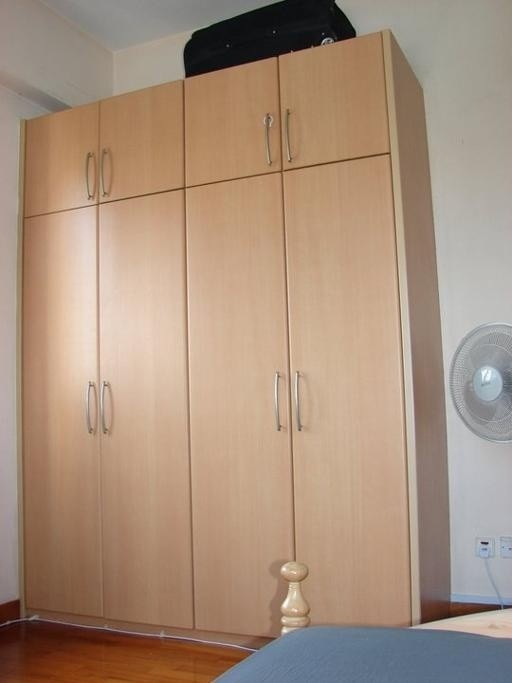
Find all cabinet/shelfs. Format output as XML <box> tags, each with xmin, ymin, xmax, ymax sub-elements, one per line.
<box><xmin>17</xmin><ymin>28</ymin><xmax>453</xmax><ymax>645</ymax></box>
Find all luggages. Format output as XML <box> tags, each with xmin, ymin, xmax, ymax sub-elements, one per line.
<box><xmin>182</xmin><ymin>0</ymin><xmax>354</xmax><ymax>76</ymax></box>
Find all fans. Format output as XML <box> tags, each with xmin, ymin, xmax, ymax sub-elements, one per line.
<box><xmin>448</xmin><ymin>320</ymin><xmax>512</xmax><ymax>445</ymax></box>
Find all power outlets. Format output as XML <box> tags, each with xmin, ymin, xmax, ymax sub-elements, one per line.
<box><xmin>476</xmin><ymin>538</ymin><xmax>495</xmax><ymax>558</ymax></box>
